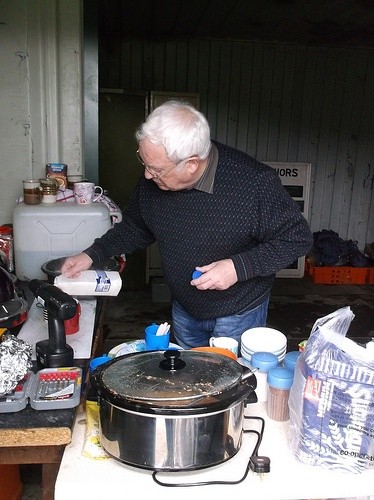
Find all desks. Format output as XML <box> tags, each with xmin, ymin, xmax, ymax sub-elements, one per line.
<box><xmin>53</xmin><ymin>358</ymin><xmax>374</xmax><ymax>500</ymax></box>
<box><xmin>0</xmin><ymin>280</ymin><xmax>107</xmax><ymax>500</ymax></box>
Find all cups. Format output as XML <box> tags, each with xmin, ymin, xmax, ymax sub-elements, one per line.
<box><xmin>73</xmin><ymin>182</ymin><xmax>102</xmax><ymax>204</ymax></box>
<box><xmin>22</xmin><ymin>179</ymin><xmax>40</xmax><ymax>205</ymax></box>
<box><xmin>145</xmin><ymin>323</ymin><xmax>170</xmax><ymax>350</ymax></box>
<box><xmin>89</xmin><ymin>354</ymin><xmax>112</xmax><ymax>372</ymax></box>
<box><xmin>209</xmin><ymin>336</ymin><xmax>238</xmax><ymax>359</ymax></box>
<box><xmin>39</xmin><ymin>178</ymin><xmax>57</xmax><ymax>203</ymax></box>
<box><xmin>67</xmin><ymin>175</ymin><xmax>88</xmax><ymax>190</ymax></box>
<box><xmin>64</xmin><ymin>303</ymin><xmax>81</xmax><ymax>336</ymax></box>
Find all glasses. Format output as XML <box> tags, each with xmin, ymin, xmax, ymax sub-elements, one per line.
<box><xmin>136</xmin><ymin>149</ymin><xmax>198</xmax><ymax>179</ymax></box>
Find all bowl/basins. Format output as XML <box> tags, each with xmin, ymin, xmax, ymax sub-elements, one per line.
<box><xmin>41</xmin><ymin>256</ymin><xmax>122</xmax><ymax>300</ymax></box>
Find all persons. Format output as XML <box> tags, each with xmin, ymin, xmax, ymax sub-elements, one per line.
<box><xmin>61</xmin><ymin>101</ymin><xmax>313</xmax><ymax>360</ymax></box>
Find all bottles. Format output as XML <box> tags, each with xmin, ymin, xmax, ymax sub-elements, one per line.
<box><xmin>250</xmin><ymin>352</ymin><xmax>279</xmax><ymax>402</ymax></box>
<box><xmin>0</xmin><ymin>223</ymin><xmax>14</xmax><ymax>273</ymax></box>
<box><xmin>283</xmin><ymin>351</ymin><xmax>302</xmax><ymax>370</ymax></box>
<box><xmin>55</xmin><ymin>271</ymin><xmax>121</xmax><ymax>297</ymax></box>
<box><xmin>265</xmin><ymin>367</ymin><xmax>294</xmax><ymax>421</ymax></box>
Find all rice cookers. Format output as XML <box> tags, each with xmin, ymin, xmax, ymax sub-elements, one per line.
<box><xmin>93</xmin><ymin>349</ymin><xmax>258</xmax><ymax>471</ymax></box>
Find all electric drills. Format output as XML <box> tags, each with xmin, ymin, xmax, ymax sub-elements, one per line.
<box><xmin>23</xmin><ymin>275</ymin><xmax>78</xmax><ymax>368</ymax></box>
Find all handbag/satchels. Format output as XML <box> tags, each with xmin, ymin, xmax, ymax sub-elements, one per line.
<box><xmin>288</xmin><ymin>306</ymin><xmax>373</xmax><ymax>473</ymax></box>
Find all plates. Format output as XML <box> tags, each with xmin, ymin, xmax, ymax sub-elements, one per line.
<box><xmin>107</xmin><ymin>339</ymin><xmax>184</xmax><ymax>359</ymax></box>
<box><xmin>240</xmin><ymin>327</ymin><xmax>287</xmax><ymax>363</ymax></box>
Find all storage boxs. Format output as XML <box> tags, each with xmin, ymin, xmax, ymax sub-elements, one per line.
<box><xmin>305</xmin><ymin>256</ymin><xmax>374</xmax><ymax>285</ymax></box>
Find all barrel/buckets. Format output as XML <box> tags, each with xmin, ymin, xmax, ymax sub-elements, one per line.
<box><xmin>13</xmin><ymin>198</ymin><xmax>112</xmax><ymax>280</ymax></box>
